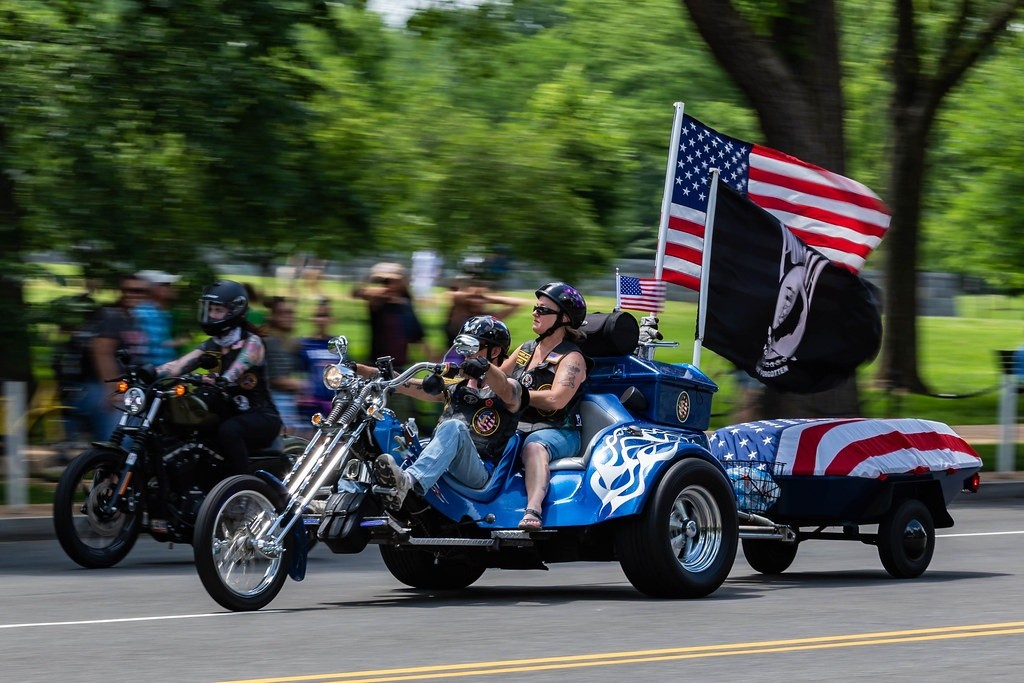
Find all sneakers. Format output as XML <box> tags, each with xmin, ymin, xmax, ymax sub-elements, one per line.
<box><xmin>373</xmin><ymin>454</ymin><xmax>414</xmax><ymax>511</ymax></box>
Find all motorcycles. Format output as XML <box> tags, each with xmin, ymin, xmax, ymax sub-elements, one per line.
<box><xmin>48</xmin><ymin>348</ymin><xmax>320</xmax><ymax>571</ymax></box>
<box><xmin>191</xmin><ymin>331</ymin><xmax>738</xmax><ymax>611</ymax></box>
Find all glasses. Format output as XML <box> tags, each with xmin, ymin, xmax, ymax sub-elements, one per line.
<box><xmin>120</xmin><ymin>288</ymin><xmax>150</xmax><ymax>296</ymax></box>
<box><xmin>533</xmin><ymin>306</ymin><xmax>560</xmax><ymax>315</ymax></box>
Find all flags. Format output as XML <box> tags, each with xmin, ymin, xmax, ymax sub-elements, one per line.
<box><xmin>616</xmin><ymin>273</ymin><xmax>667</xmax><ymax>315</ymax></box>
<box><xmin>653</xmin><ymin>101</ymin><xmax>893</xmax><ymax>293</ymax></box>
<box><xmin>695</xmin><ymin>169</ymin><xmax>885</xmax><ymax>392</ymax></box>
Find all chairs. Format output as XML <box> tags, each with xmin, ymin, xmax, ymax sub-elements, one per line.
<box><xmin>989</xmin><ymin>350</ymin><xmax>1024</xmax><ymax>395</ymax></box>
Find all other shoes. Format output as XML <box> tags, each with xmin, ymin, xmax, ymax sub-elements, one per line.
<box><xmin>151</xmin><ymin>508</ymin><xmax>180</xmax><ymax>529</ymax></box>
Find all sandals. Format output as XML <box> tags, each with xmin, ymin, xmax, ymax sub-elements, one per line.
<box><xmin>517</xmin><ymin>509</ymin><xmax>543</xmax><ymax>532</ymax></box>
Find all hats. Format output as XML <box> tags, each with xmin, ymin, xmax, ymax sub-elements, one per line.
<box><xmin>369</xmin><ymin>262</ymin><xmax>409</xmax><ymax>279</ymax></box>
<box><xmin>134</xmin><ymin>269</ymin><xmax>182</xmax><ymax>283</ymax></box>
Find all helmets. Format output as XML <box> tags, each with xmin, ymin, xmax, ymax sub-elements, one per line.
<box><xmin>193</xmin><ymin>279</ymin><xmax>250</xmax><ymax>339</ymax></box>
<box><xmin>458</xmin><ymin>315</ymin><xmax>511</xmax><ymax>355</ymax></box>
<box><xmin>535</xmin><ymin>281</ymin><xmax>587</xmax><ymax>330</ymax></box>
<box><xmin>456</xmin><ymin>255</ymin><xmax>499</xmax><ymax>282</ymax></box>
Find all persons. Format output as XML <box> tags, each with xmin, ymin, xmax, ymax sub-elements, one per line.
<box><xmin>60</xmin><ymin>278</ymin><xmax>147</xmax><ymax>452</ymax></box>
<box><xmin>151</xmin><ymin>279</ymin><xmax>283</xmax><ymax>535</ymax></box>
<box><xmin>356</xmin><ymin>262</ymin><xmax>426</xmax><ymax>371</ymax></box>
<box><xmin>356</xmin><ymin>315</ymin><xmax>530</xmax><ymax>511</ymax></box>
<box><xmin>499</xmin><ymin>283</ymin><xmax>587</xmax><ymax>531</ymax></box>
<box><xmin>445</xmin><ymin>268</ymin><xmax>519</xmax><ymax>351</ymax></box>
<box><xmin>297</xmin><ymin>301</ymin><xmax>350</xmax><ymax>422</ymax></box>
<box><xmin>257</xmin><ymin>296</ymin><xmax>314</xmax><ymax>434</ymax></box>
<box><xmin>132</xmin><ymin>270</ymin><xmax>184</xmax><ymax>380</ymax></box>
